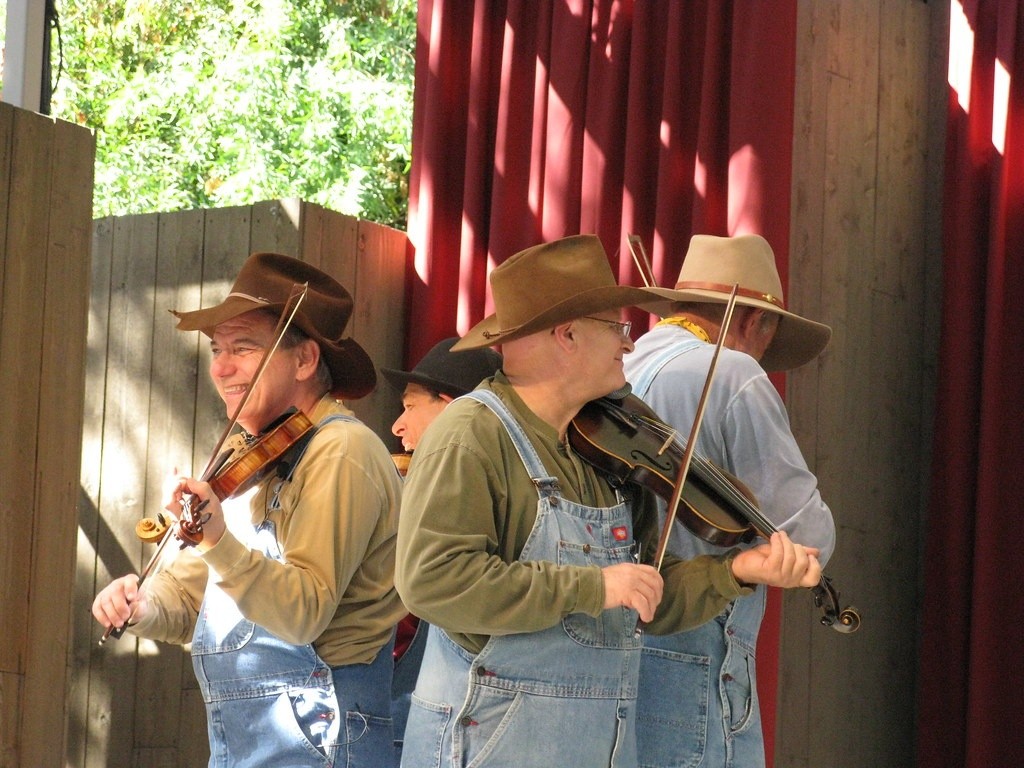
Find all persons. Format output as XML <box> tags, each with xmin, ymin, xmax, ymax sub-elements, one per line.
<box><xmin>621</xmin><ymin>234</ymin><xmax>837</xmax><ymax>768</ymax></box>
<box><xmin>382</xmin><ymin>338</ymin><xmax>504</xmax><ymax>767</ymax></box>
<box><xmin>92</xmin><ymin>252</ymin><xmax>411</xmax><ymax>768</ymax></box>
<box><xmin>393</xmin><ymin>233</ymin><xmax>821</xmax><ymax>768</ymax></box>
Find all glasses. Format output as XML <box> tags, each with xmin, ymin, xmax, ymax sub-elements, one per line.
<box><xmin>551</xmin><ymin>316</ymin><xmax>632</xmax><ymax>339</ymax></box>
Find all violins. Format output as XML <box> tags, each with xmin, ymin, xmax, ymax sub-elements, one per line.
<box><xmin>568</xmin><ymin>381</ymin><xmax>863</xmax><ymax>635</ymax></box>
<box><xmin>136</xmin><ymin>453</ymin><xmax>413</xmax><ymax>541</ymax></box>
<box><xmin>136</xmin><ymin>405</ymin><xmax>314</xmax><ymax>550</ymax></box>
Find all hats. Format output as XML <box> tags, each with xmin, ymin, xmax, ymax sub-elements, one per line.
<box><xmin>381</xmin><ymin>335</ymin><xmax>503</xmax><ymax>395</ymax></box>
<box><xmin>634</xmin><ymin>233</ymin><xmax>832</xmax><ymax>369</ymax></box>
<box><xmin>450</xmin><ymin>234</ymin><xmax>675</xmax><ymax>354</ymax></box>
<box><xmin>168</xmin><ymin>252</ymin><xmax>376</xmax><ymax>400</ymax></box>
